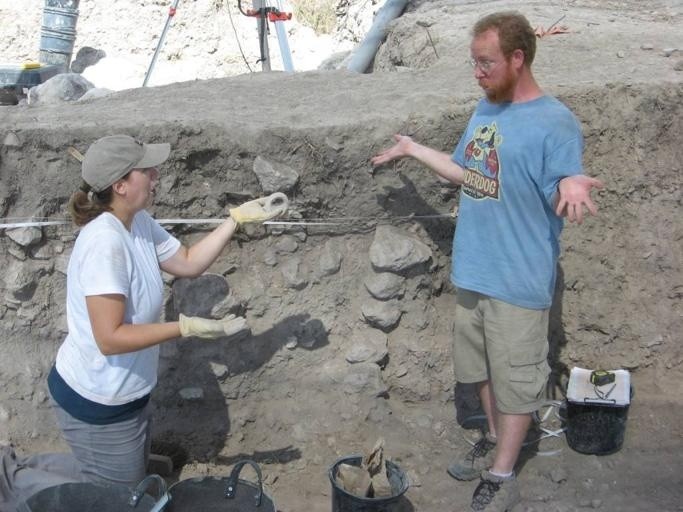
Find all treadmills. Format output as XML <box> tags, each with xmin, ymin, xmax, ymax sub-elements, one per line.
<box><xmin>590</xmin><ymin>369</ymin><xmax>615</xmax><ymax>386</ymax></box>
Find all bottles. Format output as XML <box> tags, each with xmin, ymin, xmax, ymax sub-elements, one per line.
<box><xmin>80</xmin><ymin>136</ymin><xmax>171</xmax><ymax>192</ymax></box>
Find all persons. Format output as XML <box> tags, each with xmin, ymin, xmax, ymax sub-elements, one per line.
<box><xmin>1</xmin><ymin>133</ymin><xmax>287</xmax><ymax>511</ymax></box>
<box><xmin>371</xmin><ymin>9</ymin><xmax>603</xmax><ymax>511</ymax></box>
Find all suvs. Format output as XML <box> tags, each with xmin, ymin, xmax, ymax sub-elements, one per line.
<box><xmin>564</xmin><ymin>382</ymin><xmax>634</xmax><ymax>455</ymax></box>
<box><xmin>166</xmin><ymin>461</ymin><xmax>273</xmax><ymax>512</ymax></box>
<box><xmin>38</xmin><ymin>0</ymin><xmax>79</xmax><ymax>69</ymax></box>
<box><xmin>328</xmin><ymin>455</ymin><xmax>408</xmax><ymax>512</ymax></box>
<box><xmin>17</xmin><ymin>475</ymin><xmax>165</xmax><ymax>512</ymax></box>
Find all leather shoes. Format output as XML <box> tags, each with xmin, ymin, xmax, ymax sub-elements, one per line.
<box><xmin>179</xmin><ymin>312</ymin><xmax>248</xmax><ymax>340</ymax></box>
<box><xmin>228</xmin><ymin>197</ymin><xmax>282</xmax><ymax>227</ymax></box>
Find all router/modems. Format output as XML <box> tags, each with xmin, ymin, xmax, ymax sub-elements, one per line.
<box><xmin>264</xmin><ymin>192</ymin><xmax>289</xmax><ymax>215</ymax></box>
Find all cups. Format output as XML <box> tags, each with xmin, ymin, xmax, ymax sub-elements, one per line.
<box><xmin>466</xmin><ymin>54</ymin><xmax>509</xmax><ymax>73</ymax></box>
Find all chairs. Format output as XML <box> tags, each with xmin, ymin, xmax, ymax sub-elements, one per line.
<box><xmin>466</xmin><ymin>468</ymin><xmax>522</xmax><ymax>512</ymax></box>
<box><xmin>449</xmin><ymin>431</ymin><xmax>500</xmax><ymax>479</ymax></box>
